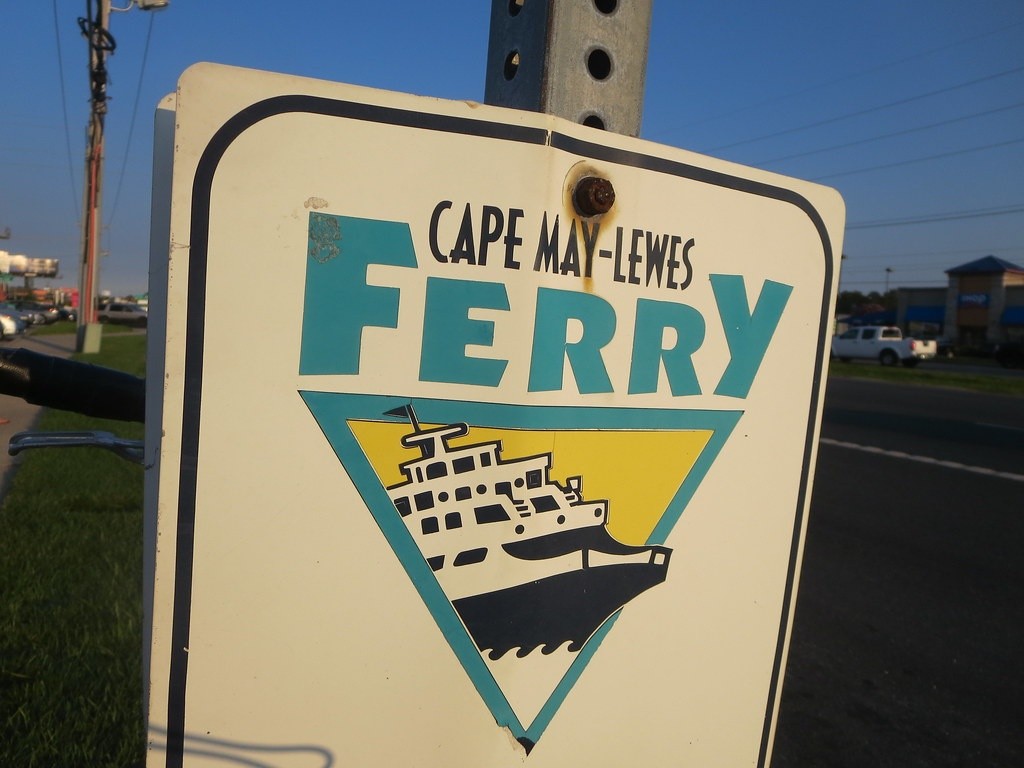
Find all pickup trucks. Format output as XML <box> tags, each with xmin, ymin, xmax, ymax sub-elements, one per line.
<box><xmin>98</xmin><ymin>299</ymin><xmax>148</xmax><ymax>328</ymax></box>
<box><xmin>828</xmin><ymin>326</ymin><xmax>938</xmax><ymax>368</ymax></box>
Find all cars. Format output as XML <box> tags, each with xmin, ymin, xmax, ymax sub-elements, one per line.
<box><xmin>1</xmin><ymin>299</ymin><xmax>76</xmax><ymax>340</ymax></box>
<box><xmin>917</xmin><ymin>332</ymin><xmax>1024</xmax><ymax>370</ymax></box>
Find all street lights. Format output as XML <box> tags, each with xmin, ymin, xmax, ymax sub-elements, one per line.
<box><xmin>884</xmin><ymin>266</ymin><xmax>891</xmax><ymax>292</ymax></box>
<box><xmin>77</xmin><ymin>0</ymin><xmax>170</xmax><ymax>349</ymax></box>
